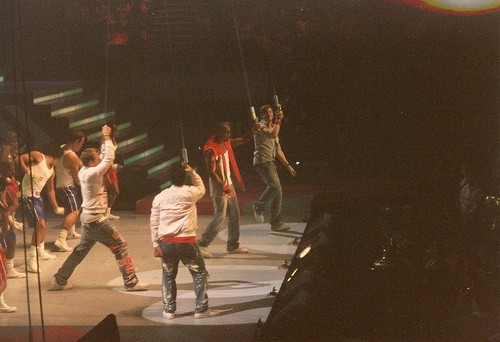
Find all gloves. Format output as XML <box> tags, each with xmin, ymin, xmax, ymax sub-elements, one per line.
<box><xmin>53</xmin><ymin>206</ymin><xmax>65</xmax><ymax>215</ymax></box>
<box><xmin>28</xmin><ymin>169</ymin><xmax>44</xmax><ymax>191</ymax></box>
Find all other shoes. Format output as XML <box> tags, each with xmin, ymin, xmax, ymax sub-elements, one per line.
<box><xmin>252</xmin><ymin>203</ymin><xmax>264</xmax><ymax>223</ymax></box>
<box><xmin>162</xmin><ymin>311</ymin><xmax>175</xmax><ymax>319</ymax></box>
<box><xmin>227</xmin><ymin>246</ymin><xmax>248</xmax><ymax>254</ymax></box>
<box><xmin>272</xmin><ymin>222</ymin><xmax>290</xmax><ymax>231</ymax></box>
<box><xmin>47</xmin><ymin>277</ymin><xmax>72</xmax><ymax>291</ymax></box>
<box><xmin>125</xmin><ymin>280</ymin><xmax>149</xmax><ymax>291</ymax></box>
<box><xmin>195</xmin><ymin>308</ymin><xmax>219</xmax><ymax>319</ymax></box>
<box><xmin>198</xmin><ymin>246</ymin><xmax>214</xmax><ymax>258</ymax></box>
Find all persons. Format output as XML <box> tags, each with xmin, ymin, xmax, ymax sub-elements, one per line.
<box><xmin>0</xmin><ymin>119</ymin><xmax>125</xmax><ymax>313</ymax></box>
<box><xmin>253</xmin><ymin>104</ymin><xmax>296</xmax><ymax>231</ymax></box>
<box><xmin>45</xmin><ymin>125</ymin><xmax>151</xmax><ymax>291</ymax></box>
<box><xmin>197</xmin><ymin>119</ymin><xmax>261</xmax><ymax>258</ymax></box>
<box><xmin>150</xmin><ymin>164</ymin><xmax>220</xmax><ymax>318</ymax></box>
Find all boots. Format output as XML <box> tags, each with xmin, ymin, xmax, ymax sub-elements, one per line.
<box><xmin>55</xmin><ymin>229</ymin><xmax>72</xmax><ymax>251</ymax></box>
<box><xmin>37</xmin><ymin>242</ymin><xmax>57</xmax><ymax>260</ymax></box>
<box><xmin>6</xmin><ymin>258</ymin><xmax>26</xmax><ymax>278</ymax></box>
<box><xmin>0</xmin><ymin>293</ymin><xmax>17</xmax><ymax>313</ymax></box>
<box><xmin>108</xmin><ymin>207</ymin><xmax>120</xmax><ymax>220</ymax></box>
<box><xmin>27</xmin><ymin>246</ymin><xmax>40</xmax><ymax>272</ymax></box>
<box><xmin>66</xmin><ymin>224</ymin><xmax>81</xmax><ymax>240</ymax></box>
